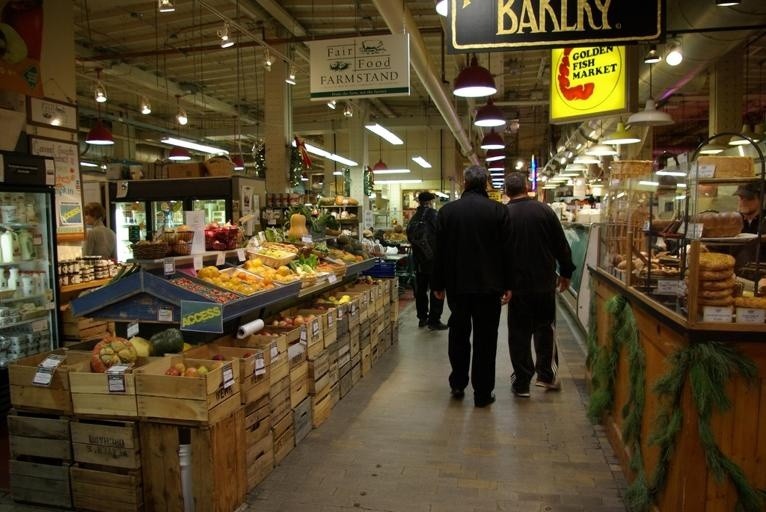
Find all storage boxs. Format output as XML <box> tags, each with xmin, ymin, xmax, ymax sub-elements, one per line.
<box><xmin>237</xmin><ymin>304</ymin><xmax>340</xmax><ymax>466</ymax></box>
<box><xmin>320</xmin><ymin>275</ymin><xmax>401</xmax><ymax>401</ymax></box>
<box><xmin>7</xmin><ymin>344</ymin><xmax>270</xmax><ymax>512</ymax></box>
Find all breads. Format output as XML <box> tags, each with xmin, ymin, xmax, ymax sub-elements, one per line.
<box><xmin>685</xmin><ymin>271</ymin><xmax>735</xmax><ymax>280</ymax></box>
<box><xmin>686</xmin><ymin>279</ymin><xmax>736</xmax><ymax>289</ymax></box>
<box><xmin>699</xmin><ymin>184</ymin><xmax>715</xmax><ymax>199</ymax></box>
<box><xmin>734</xmin><ymin>297</ymin><xmax>766</xmax><ymax>308</ymax></box>
<box><xmin>698</xmin><ymin>156</ymin><xmax>755</xmax><ymax>177</ymax></box>
<box><xmin>685</xmin><ymin>295</ymin><xmax>733</xmax><ymax>307</ymax></box>
<box><xmin>691</xmin><ymin>210</ymin><xmax>744</xmax><ymax>238</ymax></box>
<box><xmin>687</xmin><ymin>252</ymin><xmax>736</xmax><ymax>270</ymax></box>
<box><xmin>683</xmin><ymin>288</ymin><xmax>734</xmax><ymax>298</ymax></box>
<box><xmin>612</xmin><ymin>251</ymin><xmax>677</xmax><ymax>274</ymax></box>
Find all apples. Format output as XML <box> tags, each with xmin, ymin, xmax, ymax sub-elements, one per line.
<box><xmin>242</xmin><ymin>352</ymin><xmax>251</xmax><ymax>359</ymax></box>
<box><xmin>212</xmin><ymin>354</ymin><xmax>225</xmax><ymax>360</ymax></box>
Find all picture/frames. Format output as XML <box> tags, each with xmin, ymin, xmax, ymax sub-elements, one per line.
<box><xmin>26</xmin><ymin>134</ymin><xmax>87</xmax><ymax>241</ymax></box>
<box><xmin>26</xmin><ymin>94</ymin><xmax>79</xmax><ymax>133</ymax></box>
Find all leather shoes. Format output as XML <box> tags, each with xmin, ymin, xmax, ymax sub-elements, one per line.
<box><xmin>451</xmin><ymin>388</ymin><xmax>466</xmax><ymax>399</ymax></box>
<box><xmin>475</xmin><ymin>393</ymin><xmax>496</xmax><ymax>408</ymax></box>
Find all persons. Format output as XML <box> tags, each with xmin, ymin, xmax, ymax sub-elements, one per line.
<box><xmin>433</xmin><ymin>164</ymin><xmax>512</xmax><ymax>408</ymax></box>
<box><xmin>83</xmin><ymin>201</ymin><xmax>117</xmax><ymax>258</ymax></box>
<box><xmin>504</xmin><ymin>172</ymin><xmax>577</xmax><ymax>398</ymax></box>
<box><xmin>406</xmin><ymin>193</ymin><xmax>448</xmax><ymax>330</ymax></box>
<box><xmin>732</xmin><ymin>182</ymin><xmax>766</xmax><ymax>263</ymax></box>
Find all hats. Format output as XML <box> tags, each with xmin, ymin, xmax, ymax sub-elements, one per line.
<box><xmin>732</xmin><ymin>180</ymin><xmax>762</xmax><ymax>198</ymax></box>
<box><xmin>363</xmin><ymin>229</ymin><xmax>373</xmax><ymax>237</ymax></box>
<box><xmin>418</xmin><ymin>192</ymin><xmax>435</xmax><ymax>202</ymax></box>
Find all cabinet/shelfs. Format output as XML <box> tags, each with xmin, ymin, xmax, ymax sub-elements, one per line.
<box><xmin>58</xmin><ymin>277</ymin><xmax>114</xmax><ymax>351</ymax></box>
<box><xmin>71</xmin><ymin>240</ymin><xmax>382</xmax><ymax>342</ymax></box>
<box><xmin>401</xmin><ymin>188</ymin><xmax>440</xmax><ymax>233</ymax></box>
<box><xmin>556</xmin><ymin>221</ymin><xmax>601</xmax><ymax>338</ymax></box>
<box><xmin>266</xmin><ymin>205</ymin><xmax>364</xmax><ymax>246</ymax></box>
<box><xmin>588</xmin><ymin>132</ymin><xmax>766</xmax><ymax>505</ymax></box>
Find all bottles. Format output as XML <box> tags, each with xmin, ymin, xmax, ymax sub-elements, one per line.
<box><xmin>7</xmin><ymin>268</ymin><xmax>48</xmax><ymax>297</ymax></box>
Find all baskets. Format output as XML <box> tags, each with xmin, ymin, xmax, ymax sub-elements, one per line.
<box><xmin>247</xmin><ymin>250</ymin><xmax>297</xmax><ymax>266</ymax></box>
<box><xmin>205</xmin><ymin>228</ymin><xmax>239</xmax><ymax>250</ymax></box>
<box><xmin>165</xmin><ymin>231</ymin><xmax>194</xmax><ymax>255</ymax></box>
<box><xmin>130</xmin><ymin>242</ymin><xmax>168</xmax><ymax>260</ymax></box>
<box><xmin>204</xmin><ymin>158</ymin><xmax>235</xmax><ymax>176</ymax></box>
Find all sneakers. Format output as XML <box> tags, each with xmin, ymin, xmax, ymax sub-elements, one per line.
<box><xmin>428</xmin><ymin>319</ymin><xmax>448</xmax><ymax>329</ymax></box>
<box><xmin>511</xmin><ymin>380</ymin><xmax>531</xmax><ymax>397</ymax></box>
<box><xmin>418</xmin><ymin>319</ymin><xmax>429</xmax><ymax>326</ymax></box>
<box><xmin>535</xmin><ymin>375</ymin><xmax>561</xmax><ymax>389</ymax></box>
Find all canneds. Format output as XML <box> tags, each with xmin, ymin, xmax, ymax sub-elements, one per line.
<box><xmin>57</xmin><ymin>255</ymin><xmax>118</xmax><ymax>286</ymax></box>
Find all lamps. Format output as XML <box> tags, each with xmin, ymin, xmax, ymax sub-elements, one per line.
<box><xmin>624</xmin><ymin>63</ymin><xmax>675</xmax><ymax>126</ymax></box>
<box><xmin>362</xmin><ymin>122</ymin><xmax>405</xmax><ymax>147</ymax></box>
<box><xmin>584</xmin><ymin>120</ymin><xmax>618</xmax><ymax>155</ymax></box>
<box><xmin>135</xmin><ymin>91</ymin><xmax>151</xmax><ymax>114</ymax></box>
<box><xmin>263</xmin><ymin>48</ymin><xmax>272</xmax><ymax>70</ymax></box>
<box><xmin>644</xmin><ymin>42</ymin><xmax>660</xmax><ymax>63</ymax></box>
<box><xmin>488</xmin><ymin>161</ymin><xmax>506</xmax><ymax>194</ymax></box>
<box><xmin>343</xmin><ymin>102</ymin><xmax>353</xmax><ymax>116</ymax></box>
<box><xmin>50</xmin><ymin>108</ymin><xmax>65</xmax><ymax>126</ymax></box>
<box><xmin>285</xmin><ymin>64</ymin><xmax>296</xmax><ymax>85</ymax></box>
<box><xmin>514</xmin><ymin>159</ymin><xmax>524</xmax><ymax>170</ymax></box>
<box><xmin>485</xmin><ymin>150</ymin><xmax>506</xmax><ymax>161</ymax></box>
<box><xmin>435</xmin><ymin>0</ymin><xmax>448</xmax><ymax>19</ymax></box>
<box><xmin>600</xmin><ymin>114</ymin><xmax>641</xmax><ymax>145</ymax></box>
<box><xmin>174</xmin><ymin>95</ymin><xmax>188</xmax><ymax>127</ymax></box>
<box><xmin>159</xmin><ymin>0</ymin><xmax>175</xmax><ymax>12</ymax></box>
<box><xmin>452</xmin><ymin>52</ymin><xmax>498</xmax><ymax>97</ymax></box>
<box><xmin>328</xmin><ymin>100</ymin><xmax>337</xmax><ymax>110</ymax></box>
<box><xmin>666</xmin><ymin>35</ymin><xmax>683</xmax><ymax>66</ymax></box>
<box><xmin>655</xmin><ymin>157</ymin><xmax>686</xmax><ymax>177</ymax></box>
<box><xmin>480</xmin><ymin>95</ymin><xmax>505</xmax><ymax>150</ymax></box>
<box><xmin>85</xmin><ymin>70</ymin><xmax>114</xmax><ymax>144</ymax></box>
<box><xmin>167</xmin><ymin>95</ymin><xmax>192</xmax><ymax>161</ymax></box>
<box><xmin>474</xmin><ymin>52</ymin><xmax>506</xmax><ymax>127</ymax></box>
<box><xmin>536</xmin><ymin>141</ymin><xmax>594</xmax><ymax>190</ymax></box>
<box><xmin>221</xmin><ymin>23</ymin><xmax>235</xmax><ymax>48</ymax></box>
<box><xmin>291</xmin><ymin>138</ymin><xmax>358</xmax><ymax>168</ymax></box>
<box><xmin>699</xmin><ymin>142</ymin><xmax>727</xmax><ymax>155</ymax></box>
<box><xmin>232</xmin><ymin>116</ymin><xmax>245</xmax><ymax>171</ymax></box>
<box><xmin>727</xmin><ymin>42</ymin><xmax>762</xmax><ymax>144</ymax></box>
<box><xmin>373</xmin><ymin>139</ymin><xmax>387</xmax><ymax>170</ymax></box>
<box><xmin>84</xmin><ymin>0</ymin><xmax>108</xmax><ymax>102</ymax></box>
<box><xmin>161</xmin><ymin>135</ymin><xmax>229</xmax><ymax>158</ymax></box>
